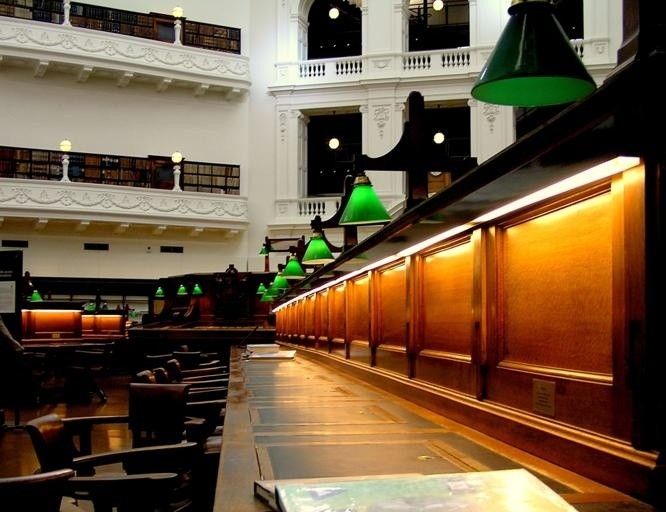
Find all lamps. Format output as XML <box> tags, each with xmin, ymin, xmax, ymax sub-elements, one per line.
<box><xmin>60</xmin><ymin>138</ymin><xmax>74</xmax><ymax>184</ymax></box>
<box><xmin>253</xmin><ymin>211</ymin><xmax>337</xmax><ymax>306</ymax></box>
<box><xmin>328</xmin><ymin>111</ymin><xmax>339</xmax><ymax>150</ymax></box>
<box><xmin>470</xmin><ymin>1</ymin><xmax>599</xmax><ymax>111</ymax></box>
<box><xmin>433</xmin><ymin>104</ymin><xmax>445</xmax><ymax>146</ymax></box>
<box><xmin>170</xmin><ymin>150</ymin><xmax>185</xmax><ymax>194</ymax></box>
<box><xmin>26</xmin><ymin>277</ymin><xmax>201</xmax><ymax>322</ymax></box>
<box><xmin>339</xmin><ymin>152</ymin><xmax>393</xmax><ymax>232</ymax></box>
<box><xmin>172</xmin><ymin>6</ymin><xmax>184</xmax><ymax>44</ymax></box>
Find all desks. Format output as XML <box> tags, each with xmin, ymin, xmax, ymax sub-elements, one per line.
<box><xmin>216</xmin><ymin>346</ymin><xmax>645</xmax><ymax>510</ymax></box>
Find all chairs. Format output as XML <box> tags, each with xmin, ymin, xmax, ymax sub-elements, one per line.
<box><xmin>64</xmin><ymin>380</ymin><xmax>204</xmax><ymax>474</ymax></box>
<box><xmin>1</xmin><ymin>468</ymin><xmax>71</xmax><ymax>511</ymax></box>
<box><xmin>24</xmin><ymin>413</ymin><xmax>196</xmax><ymax>508</ymax></box>
<box><xmin>1</xmin><ymin>319</ymin><xmax>230</xmax><ymax>464</ymax></box>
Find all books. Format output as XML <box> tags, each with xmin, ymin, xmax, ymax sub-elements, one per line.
<box><xmin>240</xmin><ymin>344</ymin><xmax>297</xmax><ymax>359</ymax></box>
<box><xmin>274</xmin><ymin>467</ymin><xmax>580</xmax><ymax>512</ymax></box>
<box><xmin>0</xmin><ymin>147</ymin><xmax>240</xmax><ymax>196</ymax></box>
<box><xmin>0</xmin><ymin>0</ymin><xmax>242</xmax><ymax>55</ymax></box>
<box><xmin>253</xmin><ymin>474</ymin><xmax>424</xmax><ymax>511</ymax></box>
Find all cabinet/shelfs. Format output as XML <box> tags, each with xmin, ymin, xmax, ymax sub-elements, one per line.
<box><xmin>0</xmin><ymin>145</ymin><xmax>240</xmax><ymax>197</ymax></box>
<box><xmin>0</xmin><ymin>1</ymin><xmax>242</xmax><ymax>56</ymax></box>
